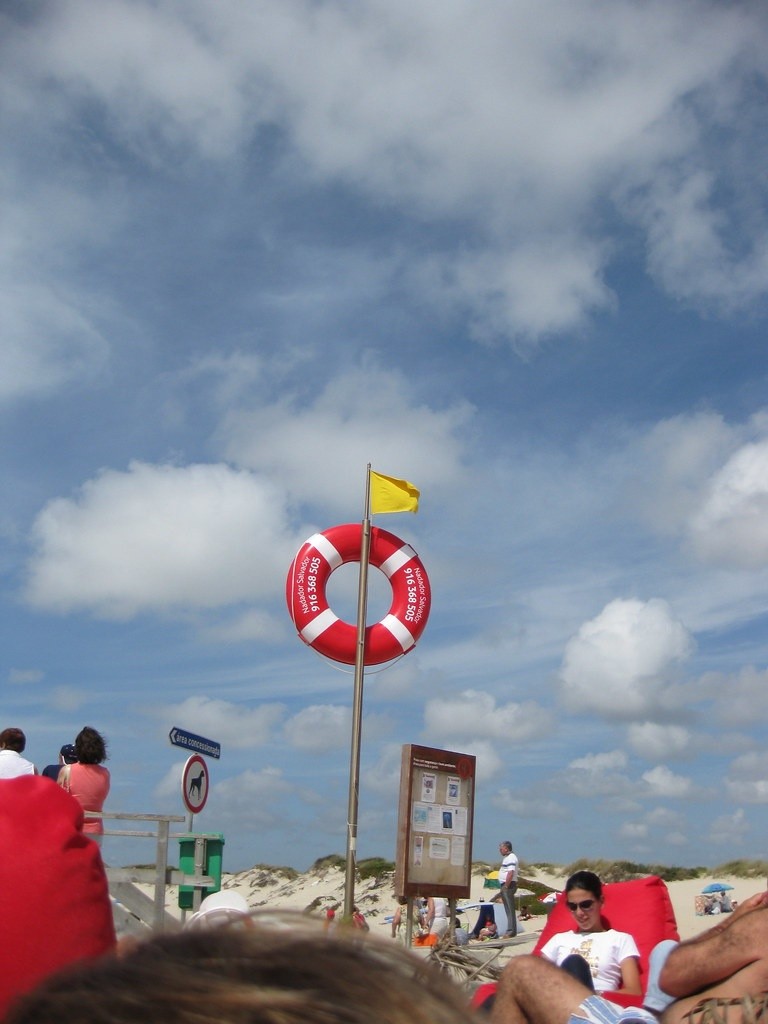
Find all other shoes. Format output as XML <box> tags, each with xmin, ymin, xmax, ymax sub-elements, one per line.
<box><xmin>499</xmin><ymin>933</ymin><xmax>516</xmax><ymax>940</ymax></box>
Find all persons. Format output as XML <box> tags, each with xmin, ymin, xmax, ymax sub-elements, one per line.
<box><xmin>42</xmin><ymin>742</ymin><xmax>74</xmax><ymax>782</ymax></box>
<box><xmin>475</xmin><ymin>870</ymin><xmax>641</xmax><ymax>1019</ymax></box>
<box><xmin>704</xmin><ymin>892</ymin><xmax>738</xmax><ymax>915</ymax></box>
<box><xmin>0</xmin><ymin>728</ymin><xmax>39</xmax><ymax>779</ymax></box>
<box><xmin>0</xmin><ymin>772</ymin><xmax>496</xmax><ymax>1024</ymax></box>
<box><xmin>489</xmin><ymin>890</ymin><xmax>768</xmax><ymax>1024</ymax></box>
<box><xmin>498</xmin><ymin>840</ymin><xmax>519</xmax><ymax>938</ymax></box>
<box><xmin>518</xmin><ymin>904</ymin><xmax>527</xmax><ymax>921</ymax></box>
<box><xmin>57</xmin><ymin>725</ymin><xmax>111</xmax><ymax>850</ymax></box>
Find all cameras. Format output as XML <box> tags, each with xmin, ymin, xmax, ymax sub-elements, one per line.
<box><xmin>60</xmin><ymin>745</ymin><xmax>79</xmax><ymax>765</ymax></box>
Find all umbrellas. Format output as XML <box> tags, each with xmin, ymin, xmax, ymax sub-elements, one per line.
<box><xmin>702</xmin><ymin>882</ymin><xmax>734</xmax><ymax>894</ymax></box>
<box><xmin>514</xmin><ymin>887</ymin><xmax>535</xmax><ymax>897</ymax></box>
<box><xmin>536</xmin><ymin>891</ymin><xmax>562</xmax><ymax>904</ymax></box>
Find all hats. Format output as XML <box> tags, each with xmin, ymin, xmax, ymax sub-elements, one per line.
<box><xmin>60</xmin><ymin>744</ymin><xmax>78</xmax><ymax>764</ymax></box>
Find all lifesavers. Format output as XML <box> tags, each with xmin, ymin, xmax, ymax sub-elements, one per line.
<box><xmin>285</xmin><ymin>523</ymin><xmax>432</xmax><ymax>668</ymax></box>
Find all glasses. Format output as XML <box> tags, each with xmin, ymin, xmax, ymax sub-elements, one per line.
<box><xmin>566</xmin><ymin>898</ymin><xmax>597</xmax><ymax>912</ymax></box>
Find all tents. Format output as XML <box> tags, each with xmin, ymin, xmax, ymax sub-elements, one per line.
<box><xmin>456</xmin><ymin>900</ymin><xmax>524</xmax><ymax>941</ymax></box>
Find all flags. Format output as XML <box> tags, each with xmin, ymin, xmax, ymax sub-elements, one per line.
<box><xmin>369</xmin><ymin>470</ymin><xmax>421</xmax><ymax>515</ymax></box>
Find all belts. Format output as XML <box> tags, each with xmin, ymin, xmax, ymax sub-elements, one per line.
<box><xmin>501</xmin><ymin>881</ymin><xmax>515</xmax><ymax>887</ymax></box>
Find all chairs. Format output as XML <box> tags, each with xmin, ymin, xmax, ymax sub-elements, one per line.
<box><xmin>469</xmin><ymin>876</ymin><xmax>680</xmax><ymax>1011</ymax></box>
